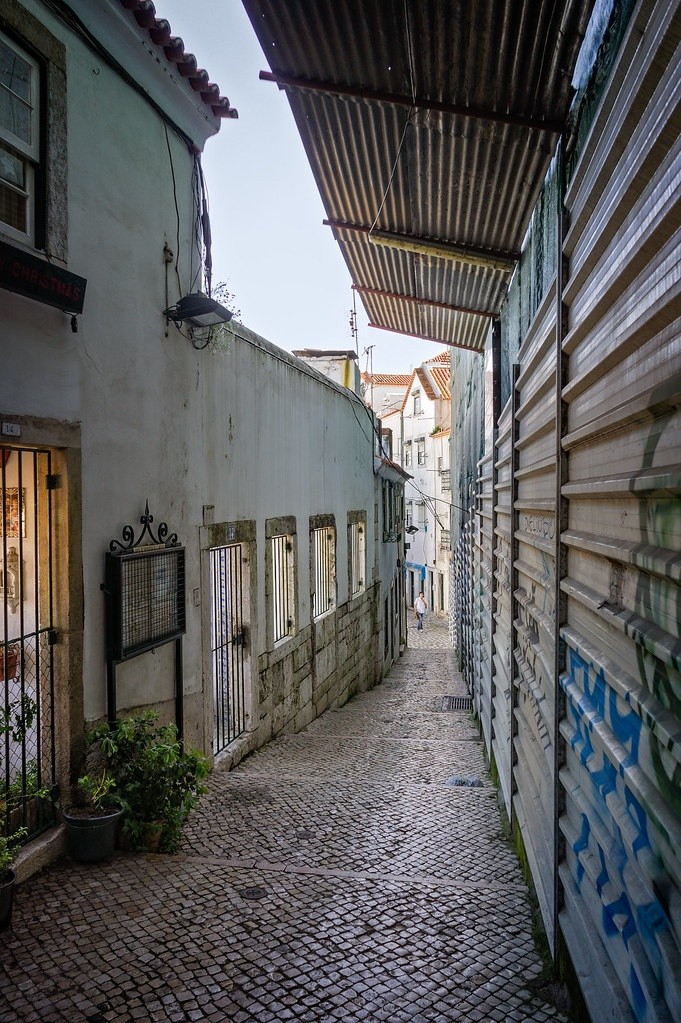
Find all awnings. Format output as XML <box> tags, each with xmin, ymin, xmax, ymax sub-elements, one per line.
<box><xmin>241</xmin><ymin>0</ymin><xmax>597</xmax><ymax>353</ymax></box>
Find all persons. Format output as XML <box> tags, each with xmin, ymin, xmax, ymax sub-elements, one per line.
<box><xmin>414</xmin><ymin>592</ymin><xmax>427</xmax><ymax>633</ymax></box>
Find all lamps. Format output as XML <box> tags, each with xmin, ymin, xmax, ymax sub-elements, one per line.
<box><xmin>405</xmin><ymin>525</ymin><xmax>419</xmax><ymax>535</ymax></box>
<box><xmin>162</xmin><ymin>297</ymin><xmax>234</xmax><ymax>327</ymax></box>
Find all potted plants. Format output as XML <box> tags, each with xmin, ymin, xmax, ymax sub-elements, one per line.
<box><xmin>0</xmin><ymin>827</ymin><xmax>28</xmax><ymax>923</ymax></box>
<box><xmin>63</xmin><ymin>706</ymin><xmax>211</xmax><ymax>863</ymax></box>
